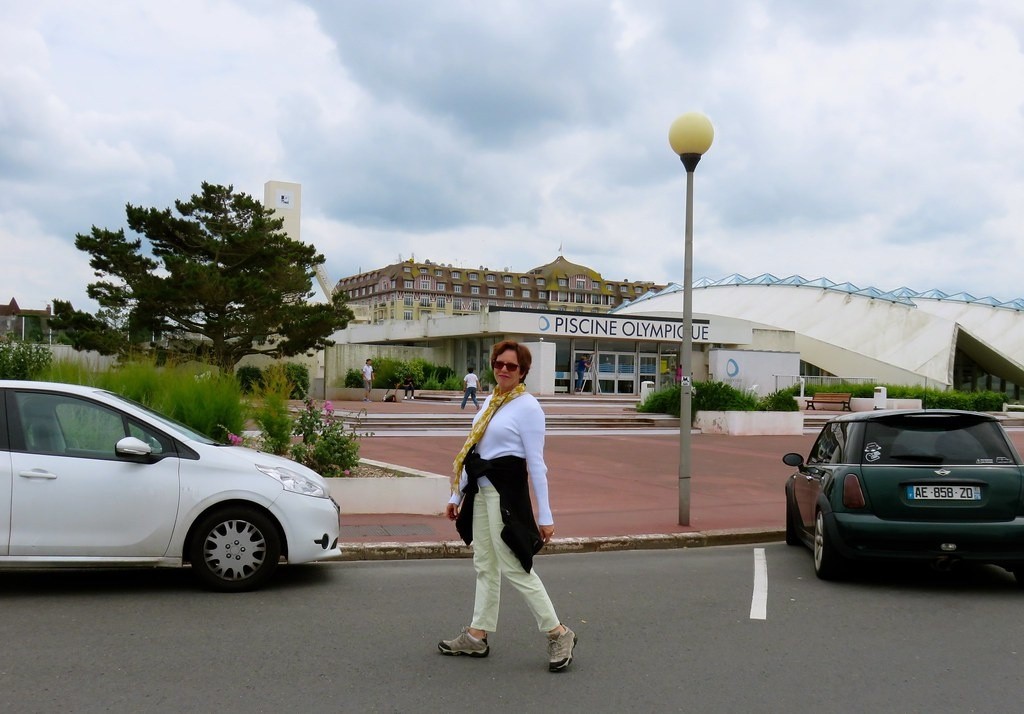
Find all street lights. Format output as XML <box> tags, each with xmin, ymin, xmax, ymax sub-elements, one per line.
<box><xmin>670</xmin><ymin>111</ymin><xmax>716</xmax><ymax>525</ymax></box>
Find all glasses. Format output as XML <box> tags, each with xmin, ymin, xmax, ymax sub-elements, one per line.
<box><xmin>492</xmin><ymin>360</ymin><xmax>520</xmax><ymax>372</ymax></box>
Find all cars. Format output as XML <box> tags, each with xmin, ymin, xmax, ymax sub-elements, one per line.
<box><xmin>782</xmin><ymin>378</ymin><xmax>1024</xmax><ymax>587</ymax></box>
<box><xmin>0</xmin><ymin>378</ymin><xmax>342</xmax><ymax>591</ymax></box>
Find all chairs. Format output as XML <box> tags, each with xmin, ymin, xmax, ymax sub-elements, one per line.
<box><xmin>890</xmin><ymin>444</ymin><xmax>911</xmax><ymax>460</ymax></box>
<box><xmin>938</xmin><ymin>436</ymin><xmax>972</xmax><ymax>462</ymax></box>
<box><xmin>24</xmin><ymin>396</ymin><xmax>63</xmax><ymax>455</ymax></box>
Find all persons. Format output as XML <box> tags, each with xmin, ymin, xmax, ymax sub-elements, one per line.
<box><xmin>363</xmin><ymin>359</ymin><xmax>375</xmax><ymax>403</ymax></box>
<box><xmin>677</xmin><ymin>365</ymin><xmax>681</xmax><ymax>383</ymax></box>
<box><xmin>403</xmin><ymin>374</ymin><xmax>415</xmax><ymax>400</ymax></box>
<box><xmin>438</xmin><ymin>340</ymin><xmax>578</xmax><ymax>672</ymax></box>
<box><xmin>575</xmin><ymin>354</ymin><xmax>591</xmax><ymax>392</ymax></box>
<box><xmin>461</xmin><ymin>367</ymin><xmax>481</xmax><ymax>410</ymax></box>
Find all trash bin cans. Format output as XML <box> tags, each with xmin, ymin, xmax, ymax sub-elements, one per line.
<box><xmin>872</xmin><ymin>386</ymin><xmax>888</xmax><ymax>411</ymax></box>
<box><xmin>639</xmin><ymin>380</ymin><xmax>655</xmax><ymax>406</ymax></box>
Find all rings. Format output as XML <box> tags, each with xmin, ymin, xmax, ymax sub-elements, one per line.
<box><xmin>552</xmin><ymin>532</ymin><xmax>554</xmax><ymax>535</ymax></box>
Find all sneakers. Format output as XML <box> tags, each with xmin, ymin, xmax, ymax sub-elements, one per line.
<box><xmin>438</xmin><ymin>627</ymin><xmax>490</xmax><ymax>658</ymax></box>
<box><xmin>545</xmin><ymin>623</ymin><xmax>578</xmax><ymax>673</ymax></box>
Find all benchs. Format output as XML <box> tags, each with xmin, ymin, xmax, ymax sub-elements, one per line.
<box><xmin>805</xmin><ymin>393</ymin><xmax>851</xmax><ymax>411</ymax></box>
